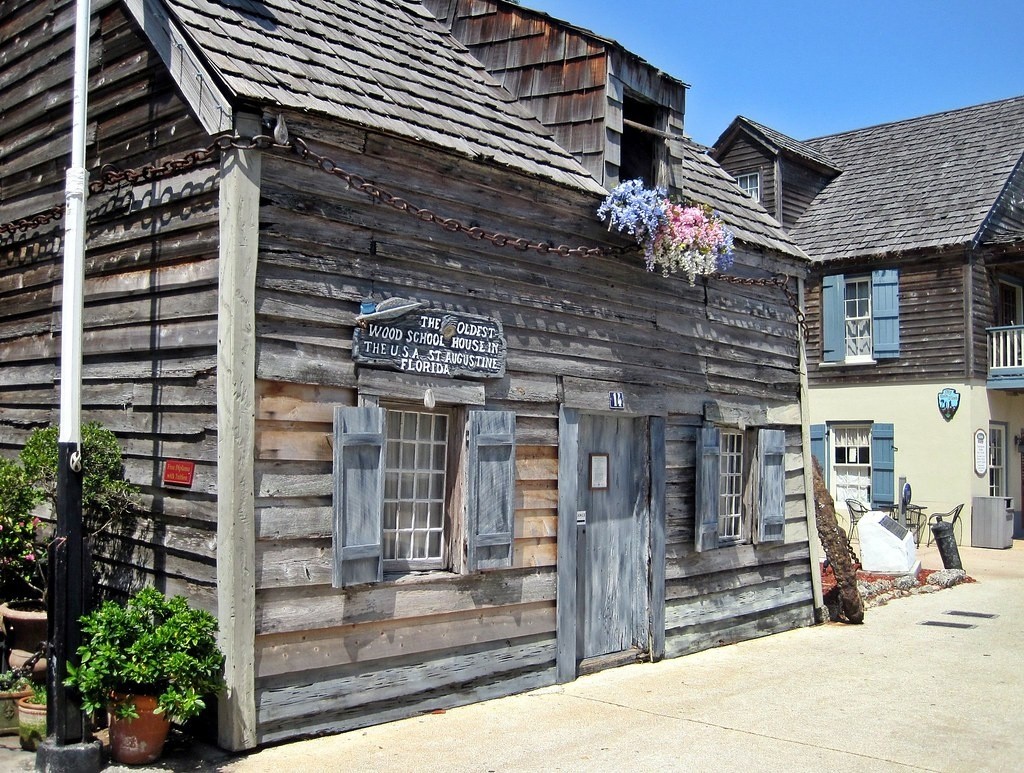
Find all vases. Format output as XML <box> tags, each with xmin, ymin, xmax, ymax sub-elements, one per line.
<box><xmin>0</xmin><ymin>600</ymin><xmax>48</xmax><ymax>685</ymax></box>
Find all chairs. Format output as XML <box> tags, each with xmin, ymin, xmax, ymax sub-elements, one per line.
<box><xmin>889</xmin><ymin>503</ymin><xmax>926</xmax><ymax>550</ymax></box>
<box><xmin>846</xmin><ymin>499</ymin><xmax>879</xmax><ymax>543</ymax></box>
<box><xmin>927</xmin><ymin>504</ymin><xmax>965</xmax><ymax>547</ymax></box>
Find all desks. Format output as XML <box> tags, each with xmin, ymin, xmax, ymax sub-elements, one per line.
<box><xmin>879</xmin><ymin>504</ymin><xmax>927</xmax><ymax>549</ymax></box>
<box><xmin>972</xmin><ymin>497</ymin><xmax>1014</xmax><ymax>548</ymax></box>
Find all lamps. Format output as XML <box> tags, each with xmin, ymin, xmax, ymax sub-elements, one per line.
<box><xmin>1015</xmin><ymin>434</ymin><xmax>1024</xmax><ymax>452</ymax></box>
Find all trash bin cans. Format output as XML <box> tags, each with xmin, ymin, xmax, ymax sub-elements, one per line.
<box><xmin>970</xmin><ymin>495</ymin><xmax>1016</xmax><ymax>550</ymax></box>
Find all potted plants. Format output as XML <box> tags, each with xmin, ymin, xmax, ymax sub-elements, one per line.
<box><xmin>61</xmin><ymin>585</ymin><xmax>228</xmax><ymax>763</ymax></box>
<box><xmin>0</xmin><ymin>674</ymin><xmax>47</xmax><ymax>752</ymax></box>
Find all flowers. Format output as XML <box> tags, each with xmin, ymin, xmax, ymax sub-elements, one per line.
<box><xmin>0</xmin><ymin>513</ymin><xmax>60</xmax><ymax>609</ymax></box>
